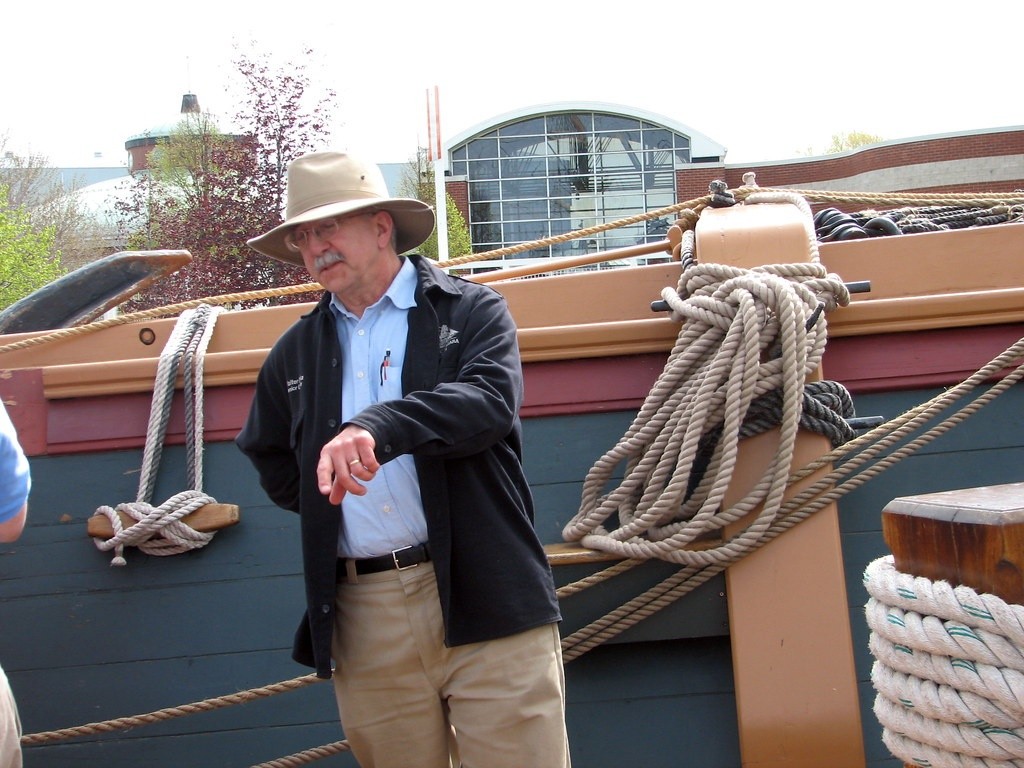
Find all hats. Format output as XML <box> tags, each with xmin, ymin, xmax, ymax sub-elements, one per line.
<box><xmin>245</xmin><ymin>153</ymin><xmax>436</xmax><ymax>268</ymax></box>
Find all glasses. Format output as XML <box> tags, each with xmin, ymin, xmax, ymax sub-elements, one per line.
<box><xmin>284</xmin><ymin>211</ymin><xmax>378</xmax><ymax>253</ymax></box>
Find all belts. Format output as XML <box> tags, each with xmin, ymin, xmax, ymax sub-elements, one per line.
<box><xmin>335</xmin><ymin>542</ymin><xmax>432</xmax><ymax>577</ymax></box>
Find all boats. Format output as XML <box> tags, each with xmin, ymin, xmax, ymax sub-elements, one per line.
<box><xmin>1</xmin><ymin>168</ymin><xmax>1024</xmax><ymax>768</ymax></box>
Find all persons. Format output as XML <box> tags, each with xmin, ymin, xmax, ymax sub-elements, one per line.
<box><xmin>235</xmin><ymin>152</ymin><xmax>572</xmax><ymax>768</ymax></box>
<box><xmin>0</xmin><ymin>397</ymin><xmax>32</xmax><ymax>768</ymax></box>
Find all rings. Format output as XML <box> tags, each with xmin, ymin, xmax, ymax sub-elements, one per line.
<box><xmin>348</xmin><ymin>459</ymin><xmax>360</xmax><ymax>466</ymax></box>
